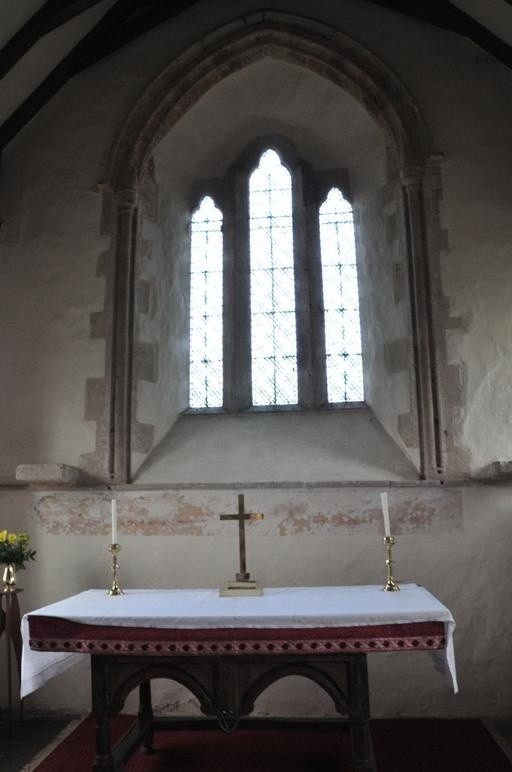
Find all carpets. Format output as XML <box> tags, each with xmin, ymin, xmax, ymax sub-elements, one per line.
<box><xmin>0</xmin><ymin>711</ymin><xmax>79</xmax><ymax>772</ymax></box>
<box><xmin>29</xmin><ymin>714</ymin><xmax>512</xmax><ymax>771</ymax></box>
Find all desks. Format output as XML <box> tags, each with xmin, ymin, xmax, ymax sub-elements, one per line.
<box><xmin>18</xmin><ymin>579</ymin><xmax>464</xmax><ymax>772</ymax></box>
<box><xmin>2</xmin><ymin>590</ymin><xmax>23</xmax><ymax>744</ymax></box>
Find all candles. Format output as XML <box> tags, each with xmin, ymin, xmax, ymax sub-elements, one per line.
<box><xmin>378</xmin><ymin>488</ymin><xmax>393</xmax><ymax>539</ymax></box>
<box><xmin>108</xmin><ymin>495</ymin><xmax>120</xmax><ymax>543</ymax></box>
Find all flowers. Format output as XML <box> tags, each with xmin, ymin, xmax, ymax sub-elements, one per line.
<box><xmin>0</xmin><ymin>529</ymin><xmax>37</xmax><ymax>570</ymax></box>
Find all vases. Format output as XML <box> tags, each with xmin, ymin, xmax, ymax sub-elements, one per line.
<box><xmin>3</xmin><ymin>564</ymin><xmax>16</xmax><ymax>592</ymax></box>
